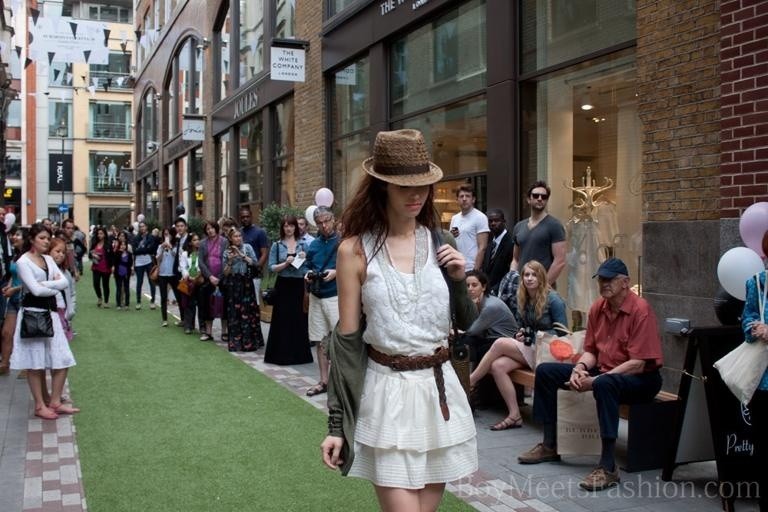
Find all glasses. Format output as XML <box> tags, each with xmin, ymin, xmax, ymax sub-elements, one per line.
<box><xmin>531</xmin><ymin>193</ymin><xmax>548</xmax><ymax>200</ymax></box>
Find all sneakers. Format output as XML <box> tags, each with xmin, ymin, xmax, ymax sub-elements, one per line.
<box><xmin>149</xmin><ymin>265</ymin><xmax>159</xmax><ymax>280</ymax></box>
<box><xmin>177</xmin><ymin>279</ymin><xmax>190</xmax><ymax>295</ymax></box>
<box><xmin>579</xmin><ymin>465</ymin><xmax>621</xmax><ymax>491</ymax></box>
<box><xmin>210</xmin><ymin>285</ymin><xmax>223</xmax><ymax>317</ymax></box>
<box><xmin>161</xmin><ymin>321</ymin><xmax>229</xmax><ymax>341</ymax></box>
<box><xmin>517</xmin><ymin>442</ymin><xmax>561</xmax><ymax>464</ymax></box>
<box><xmin>96</xmin><ymin>297</ymin><xmax>156</xmax><ymax>311</ymax></box>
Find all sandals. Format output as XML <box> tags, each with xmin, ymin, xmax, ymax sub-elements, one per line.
<box><xmin>470</xmin><ymin>386</ymin><xmax>475</xmax><ymax>396</ymax></box>
<box><xmin>306</xmin><ymin>381</ymin><xmax>328</xmax><ymax>396</ymax></box>
<box><xmin>0</xmin><ymin>365</ymin><xmax>80</xmax><ymax>419</ymax></box>
<box><xmin>490</xmin><ymin>416</ymin><xmax>523</xmax><ymax>431</ymax></box>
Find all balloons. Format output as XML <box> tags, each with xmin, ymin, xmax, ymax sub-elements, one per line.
<box><xmin>739</xmin><ymin>201</ymin><xmax>768</xmax><ymax>258</ymax></box>
<box><xmin>716</xmin><ymin>246</ymin><xmax>765</xmax><ymax>301</ymax></box>
<box><xmin>315</xmin><ymin>187</ymin><xmax>334</xmax><ymax>208</ymax></box>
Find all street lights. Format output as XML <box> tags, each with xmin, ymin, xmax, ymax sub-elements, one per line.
<box><xmin>57</xmin><ymin>119</ymin><xmax>70</xmax><ymax>223</ymax></box>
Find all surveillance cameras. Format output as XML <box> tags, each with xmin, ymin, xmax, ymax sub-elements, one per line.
<box><xmin>147</xmin><ymin>142</ymin><xmax>154</xmax><ymax>150</ymax></box>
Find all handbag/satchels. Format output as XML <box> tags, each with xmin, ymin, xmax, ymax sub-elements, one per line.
<box><xmin>20</xmin><ymin>309</ymin><xmax>54</xmax><ymax>338</ymax></box>
<box><xmin>262</xmin><ymin>288</ymin><xmax>278</xmax><ymax>306</ymax></box>
<box><xmin>430</xmin><ymin>228</ymin><xmax>470</xmax><ymax>398</ymax></box>
<box><xmin>71</xmin><ymin>238</ymin><xmax>87</xmax><ymax>261</ymax></box>
<box><xmin>712</xmin><ymin>269</ymin><xmax>768</xmax><ymax>406</ymax></box>
<box><xmin>557</xmin><ymin>384</ymin><xmax>604</xmax><ymax>455</ymax></box>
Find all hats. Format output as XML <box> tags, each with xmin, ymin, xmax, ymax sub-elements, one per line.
<box><xmin>592</xmin><ymin>257</ymin><xmax>628</xmax><ymax>279</ymax></box>
<box><xmin>362</xmin><ymin>129</ymin><xmax>444</xmax><ymax>187</ymax></box>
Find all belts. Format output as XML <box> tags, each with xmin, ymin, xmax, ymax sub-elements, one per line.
<box><xmin>368</xmin><ymin>344</ymin><xmax>450</xmax><ymax>421</ymax></box>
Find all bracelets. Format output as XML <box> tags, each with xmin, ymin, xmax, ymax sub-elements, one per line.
<box><xmin>576</xmin><ymin>362</ymin><xmax>588</xmax><ymax>371</ymax></box>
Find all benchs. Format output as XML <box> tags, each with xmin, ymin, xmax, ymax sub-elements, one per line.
<box><xmin>507</xmin><ymin>365</ymin><xmax>677</xmax><ymax>475</ymax></box>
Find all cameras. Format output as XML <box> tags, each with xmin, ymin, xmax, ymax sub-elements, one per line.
<box><xmin>307</xmin><ymin>271</ymin><xmax>327</xmax><ymax>294</ymax></box>
<box><xmin>520</xmin><ymin>327</ymin><xmax>534</xmax><ymax>346</ymax></box>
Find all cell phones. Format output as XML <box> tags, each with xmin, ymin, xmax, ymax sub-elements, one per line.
<box><xmin>164</xmin><ymin>236</ymin><xmax>170</xmax><ymax>245</ymax></box>
<box><xmin>230</xmin><ymin>246</ymin><xmax>236</xmax><ymax>255</ymax></box>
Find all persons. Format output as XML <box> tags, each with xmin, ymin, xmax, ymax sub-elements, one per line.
<box><xmin>97</xmin><ymin>162</ymin><xmax>106</xmax><ymax>188</ymax></box>
<box><xmin>481</xmin><ymin>208</ymin><xmax>514</xmax><ymax>296</ymax></box>
<box><xmin>740</xmin><ymin>228</ymin><xmax>768</xmax><ymax>512</ymax></box>
<box><xmin>449</xmin><ymin>183</ymin><xmax>492</xmax><ymax>275</ymax></box>
<box><xmin>517</xmin><ymin>257</ymin><xmax>665</xmax><ymax>492</ymax></box>
<box><xmin>509</xmin><ymin>179</ymin><xmax>566</xmax><ymax>291</ymax></box>
<box><xmin>319</xmin><ymin>129</ymin><xmax>480</xmax><ymax>512</ymax></box>
<box><xmin>107</xmin><ymin>159</ymin><xmax>118</xmax><ymax>186</ymax></box>
<box><xmin>469</xmin><ymin>259</ymin><xmax>568</xmax><ymax>431</ymax></box>
<box><xmin>448</xmin><ymin>267</ymin><xmax>518</xmax><ymax>363</ymax></box>
